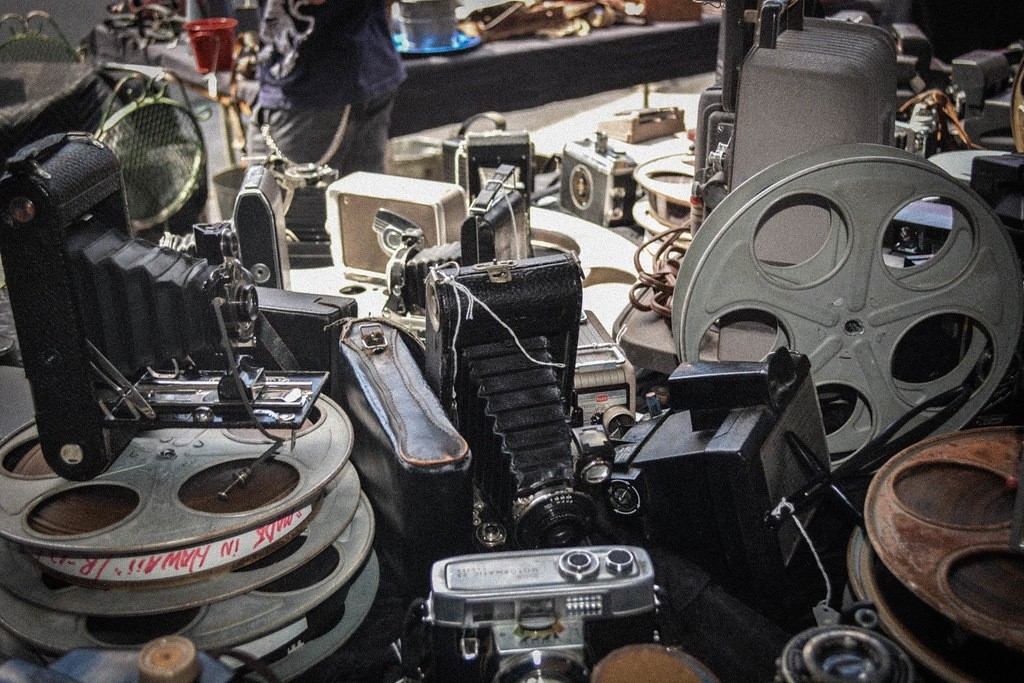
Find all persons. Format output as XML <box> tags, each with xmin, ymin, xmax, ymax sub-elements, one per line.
<box><xmin>234</xmin><ymin>0</ymin><xmax>407</xmax><ymax>190</ymax></box>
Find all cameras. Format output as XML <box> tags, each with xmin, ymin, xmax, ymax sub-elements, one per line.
<box><xmin>558</xmin><ymin>131</ymin><xmax>638</xmax><ymax>232</ymax></box>
<box><xmin>763</xmin><ymin>600</ymin><xmax>915</xmax><ymax>683</ymax></box>
<box><xmin>410</xmin><ymin>546</ymin><xmax>659</xmax><ymax>683</ymax></box>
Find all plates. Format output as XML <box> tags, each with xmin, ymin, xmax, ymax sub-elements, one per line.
<box><xmin>392</xmin><ymin>32</ymin><xmax>482</xmax><ymax>56</ymax></box>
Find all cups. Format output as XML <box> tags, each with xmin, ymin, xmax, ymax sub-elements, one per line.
<box><xmin>399</xmin><ymin>0</ymin><xmax>457</xmax><ymax>48</ymax></box>
<box><xmin>182</xmin><ymin>17</ymin><xmax>239</xmax><ymax>75</ymax></box>
